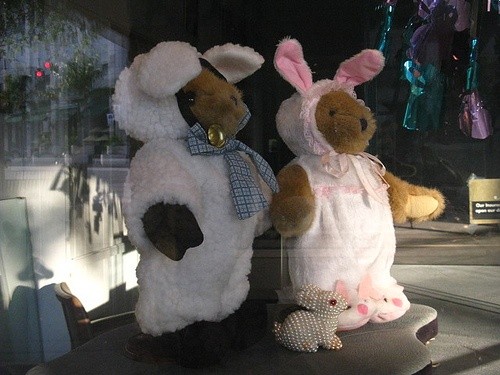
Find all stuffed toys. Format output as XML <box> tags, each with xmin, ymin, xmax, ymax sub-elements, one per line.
<box><xmin>268</xmin><ymin>35</ymin><xmax>447</xmax><ymax>333</ymax></box>
<box><xmin>272</xmin><ymin>283</ymin><xmax>352</xmax><ymax>355</ymax></box>
<box><xmin>110</xmin><ymin>39</ymin><xmax>282</xmax><ymax>370</ymax></box>
